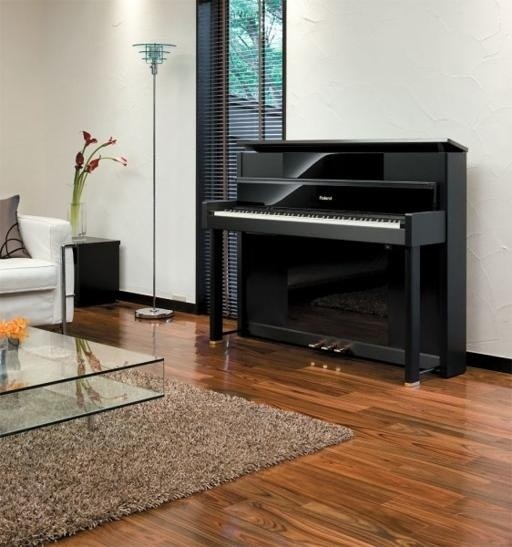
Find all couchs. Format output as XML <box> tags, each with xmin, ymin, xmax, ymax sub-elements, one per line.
<box><xmin>0</xmin><ymin>194</ymin><xmax>73</xmax><ymax>334</ymax></box>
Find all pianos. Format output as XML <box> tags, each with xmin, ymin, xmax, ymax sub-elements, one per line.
<box><xmin>201</xmin><ymin>138</ymin><xmax>468</xmax><ymax>385</ymax></box>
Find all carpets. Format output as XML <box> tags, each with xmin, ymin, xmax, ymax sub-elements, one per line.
<box><xmin>1</xmin><ymin>332</ymin><xmax>355</xmax><ymax>546</ymax></box>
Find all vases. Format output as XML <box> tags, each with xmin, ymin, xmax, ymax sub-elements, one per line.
<box><xmin>69</xmin><ymin>130</ymin><xmax>129</xmax><ymax>240</ymax></box>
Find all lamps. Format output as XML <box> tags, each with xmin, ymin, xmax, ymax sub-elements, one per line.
<box><xmin>130</xmin><ymin>43</ymin><xmax>177</xmax><ymax>320</ymax></box>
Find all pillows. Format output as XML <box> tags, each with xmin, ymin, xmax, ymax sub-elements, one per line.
<box><xmin>0</xmin><ymin>195</ymin><xmax>34</xmax><ymax>258</ymax></box>
